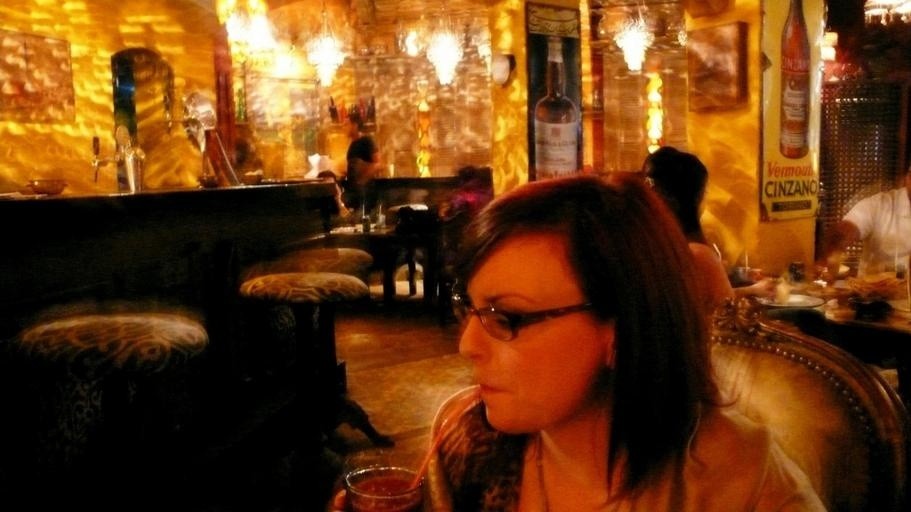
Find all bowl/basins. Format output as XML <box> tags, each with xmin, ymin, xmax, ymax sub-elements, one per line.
<box><xmin>524</xmin><ymin>438</ymin><xmax>626</xmax><ymax>512</ymax></box>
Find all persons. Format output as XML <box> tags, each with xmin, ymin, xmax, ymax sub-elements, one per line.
<box><xmin>640</xmin><ymin>143</ymin><xmax>787</xmax><ymax>313</ymax></box>
<box><xmin>317</xmin><ymin>170</ymin><xmax>353</xmax><ymax>228</ymax></box>
<box><xmin>438</xmin><ymin>162</ymin><xmax>496</xmax><ymax>327</ymax></box>
<box><xmin>819</xmin><ymin>149</ymin><xmax>911</xmax><ymax>280</ymax></box>
<box><xmin>342</xmin><ymin>112</ymin><xmax>384</xmax><ymax>185</ymax></box>
<box><xmin>326</xmin><ymin>169</ymin><xmax>830</xmax><ymax>512</ymax></box>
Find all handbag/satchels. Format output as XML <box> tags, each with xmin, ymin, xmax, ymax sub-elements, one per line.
<box><xmin>336</xmin><ymin>202</ymin><xmax>345</xmax><ymax>208</ymax></box>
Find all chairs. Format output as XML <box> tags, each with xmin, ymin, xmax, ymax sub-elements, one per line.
<box><xmin>329</xmin><ymin>193</ymin><xmax>479</xmax><ymax>332</ymax></box>
<box><xmin>704</xmin><ymin>292</ymin><xmax>909</xmax><ymax>510</ymax></box>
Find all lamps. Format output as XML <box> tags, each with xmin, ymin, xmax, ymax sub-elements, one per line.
<box><xmin>610</xmin><ymin>0</ymin><xmax>658</xmax><ymax>74</ymax></box>
<box><xmin>301</xmin><ymin>0</ymin><xmax>349</xmax><ymax>90</ymax></box>
<box><xmin>704</xmin><ymin>292</ymin><xmax>909</xmax><ymax>510</ymax></box>
<box><xmin>423</xmin><ymin>0</ymin><xmax>466</xmax><ymax>87</ymax></box>
<box><xmin>862</xmin><ymin>0</ymin><xmax>911</xmax><ymax>29</ymax></box>
<box><xmin>329</xmin><ymin>193</ymin><xmax>479</xmax><ymax>332</ymax></box>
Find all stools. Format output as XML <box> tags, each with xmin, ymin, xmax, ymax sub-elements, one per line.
<box><xmin>10</xmin><ymin>234</ymin><xmax>403</xmax><ymax>510</ymax></box>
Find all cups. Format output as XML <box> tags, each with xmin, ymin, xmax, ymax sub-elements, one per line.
<box><xmin>451</xmin><ymin>291</ymin><xmax>630</xmax><ymax>341</ymax></box>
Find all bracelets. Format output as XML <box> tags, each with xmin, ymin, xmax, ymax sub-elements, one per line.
<box><xmin>242</xmin><ymin>175</ymin><xmax>263</xmax><ymax>184</ymax></box>
<box><xmin>27</xmin><ymin>178</ymin><xmax>66</xmax><ymax>196</ymax></box>
<box><xmin>199</xmin><ymin>175</ymin><xmax>224</xmax><ymax>188</ymax></box>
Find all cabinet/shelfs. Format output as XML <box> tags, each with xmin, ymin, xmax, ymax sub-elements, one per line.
<box><xmin>328</xmin><ymin>92</ymin><xmax>376</xmax><ymax>125</ymax></box>
<box><xmin>778</xmin><ymin>0</ymin><xmax>813</xmax><ymax>160</ymax></box>
<box><xmin>534</xmin><ymin>35</ymin><xmax>581</xmax><ymax>182</ymax></box>
<box><xmin>235</xmin><ymin>87</ymin><xmax>246</xmax><ymax>120</ymax></box>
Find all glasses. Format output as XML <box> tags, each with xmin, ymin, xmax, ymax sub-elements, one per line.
<box><xmin>423</xmin><ymin>0</ymin><xmax>466</xmax><ymax>87</ymax></box>
<box><xmin>301</xmin><ymin>0</ymin><xmax>349</xmax><ymax>90</ymax></box>
<box><xmin>862</xmin><ymin>0</ymin><xmax>911</xmax><ymax>29</ymax></box>
<box><xmin>610</xmin><ymin>0</ymin><xmax>658</xmax><ymax>74</ymax></box>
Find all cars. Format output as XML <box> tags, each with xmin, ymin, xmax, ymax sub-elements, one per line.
<box><xmin>122</xmin><ymin>156</ymin><xmax>146</xmax><ymax>192</ymax></box>
<box><xmin>361</xmin><ymin>213</ymin><xmax>385</xmax><ymax>232</ymax></box>
<box><xmin>342</xmin><ymin>463</ymin><xmax>426</xmax><ymax>512</ymax></box>
<box><xmin>735</xmin><ymin>266</ymin><xmax>754</xmax><ymax>286</ymax></box>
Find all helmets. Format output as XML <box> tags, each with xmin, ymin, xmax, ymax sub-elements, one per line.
<box><xmin>756</xmin><ymin>293</ymin><xmax>824</xmax><ymax>307</ymax></box>
<box><xmin>822</xmin><ymin>264</ymin><xmax>849</xmax><ymax>274</ymax></box>
